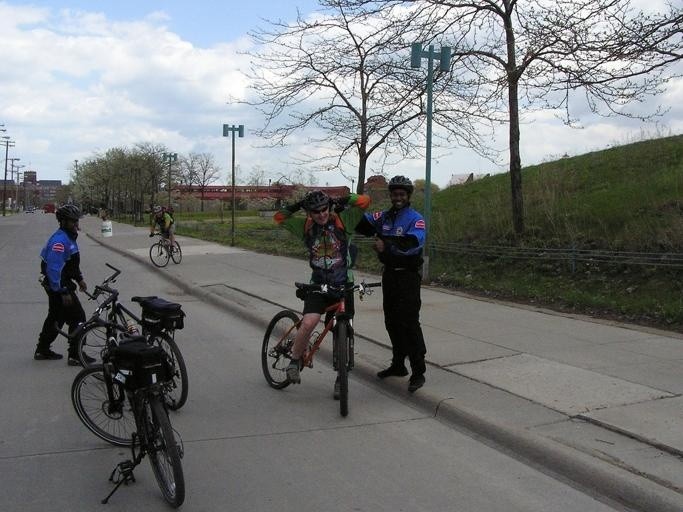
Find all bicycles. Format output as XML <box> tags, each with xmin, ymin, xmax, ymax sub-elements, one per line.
<box><xmin>53</xmin><ymin>262</ymin><xmax>188</xmax><ymax>508</ymax></box>
<box><xmin>149</xmin><ymin>231</ymin><xmax>181</xmax><ymax>268</ymax></box>
<box><xmin>259</xmin><ymin>280</ymin><xmax>382</xmax><ymax>416</ymax></box>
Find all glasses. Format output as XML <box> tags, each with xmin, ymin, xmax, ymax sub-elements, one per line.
<box><xmin>312</xmin><ymin>207</ymin><xmax>327</xmax><ymax>213</ymax></box>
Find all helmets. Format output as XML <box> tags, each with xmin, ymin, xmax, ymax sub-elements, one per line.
<box><xmin>388</xmin><ymin>176</ymin><xmax>414</xmax><ymax>193</ymax></box>
<box><xmin>302</xmin><ymin>190</ymin><xmax>329</xmax><ymax>209</ymax></box>
<box><xmin>154</xmin><ymin>206</ymin><xmax>162</xmax><ymax>213</ymax></box>
<box><xmin>56</xmin><ymin>205</ymin><xmax>81</xmax><ymax>222</ymax></box>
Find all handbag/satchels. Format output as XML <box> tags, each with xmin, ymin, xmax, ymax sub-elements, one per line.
<box><xmin>112</xmin><ymin>340</ymin><xmax>167</xmax><ymax>391</ymax></box>
<box><xmin>143</xmin><ymin>298</ymin><xmax>185</xmax><ymax>332</ymax></box>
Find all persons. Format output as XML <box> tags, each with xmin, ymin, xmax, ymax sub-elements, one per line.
<box><xmin>275</xmin><ymin>189</ymin><xmax>371</xmax><ymax>400</ymax></box>
<box><xmin>149</xmin><ymin>204</ymin><xmax>176</xmax><ymax>257</ymax></box>
<box><xmin>335</xmin><ymin>176</ymin><xmax>427</xmax><ymax>392</ymax></box>
<box><xmin>34</xmin><ymin>204</ymin><xmax>97</xmax><ymax>366</ymax></box>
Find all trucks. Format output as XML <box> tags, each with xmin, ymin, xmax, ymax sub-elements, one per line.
<box><xmin>42</xmin><ymin>204</ymin><xmax>54</xmax><ymax>212</ymax></box>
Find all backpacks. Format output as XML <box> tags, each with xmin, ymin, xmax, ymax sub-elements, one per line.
<box><xmin>162</xmin><ymin>206</ymin><xmax>174</xmax><ymax>215</ymax></box>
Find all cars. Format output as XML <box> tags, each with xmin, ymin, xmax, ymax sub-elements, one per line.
<box><xmin>25</xmin><ymin>206</ymin><xmax>33</xmax><ymax>214</ymax></box>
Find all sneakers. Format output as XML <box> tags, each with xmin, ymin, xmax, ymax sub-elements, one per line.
<box><xmin>408</xmin><ymin>375</ymin><xmax>425</xmax><ymax>392</ymax></box>
<box><xmin>378</xmin><ymin>367</ymin><xmax>407</xmax><ymax>377</ymax></box>
<box><xmin>34</xmin><ymin>350</ymin><xmax>62</xmax><ymax>360</ymax></box>
<box><xmin>286</xmin><ymin>360</ymin><xmax>302</xmax><ymax>383</ymax></box>
<box><xmin>68</xmin><ymin>355</ymin><xmax>96</xmax><ymax>366</ymax></box>
<box><xmin>334</xmin><ymin>377</ymin><xmax>341</xmax><ymax>400</ymax></box>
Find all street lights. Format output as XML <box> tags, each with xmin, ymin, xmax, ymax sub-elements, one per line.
<box><xmin>411</xmin><ymin>42</ymin><xmax>450</xmax><ymax>254</ymax></box>
<box><xmin>223</xmin><ymin>124</ymin><xmax>244</xmax><ymax>241</ymax></box>
<box><xmin>0</xmin><ymin>124</ymin><xmax>26</xmax><ymax>211</ymax></box>
<box><xmin>162</xmin><ymin>152</ymin><xmax>177</xmax><ymax>213</ymax></box>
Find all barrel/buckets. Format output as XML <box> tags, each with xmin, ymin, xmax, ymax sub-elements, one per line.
<box><xmin>101</xmin><ymin>220</ymin><xmax>113</xmax><ymax>237</ymax></box>
